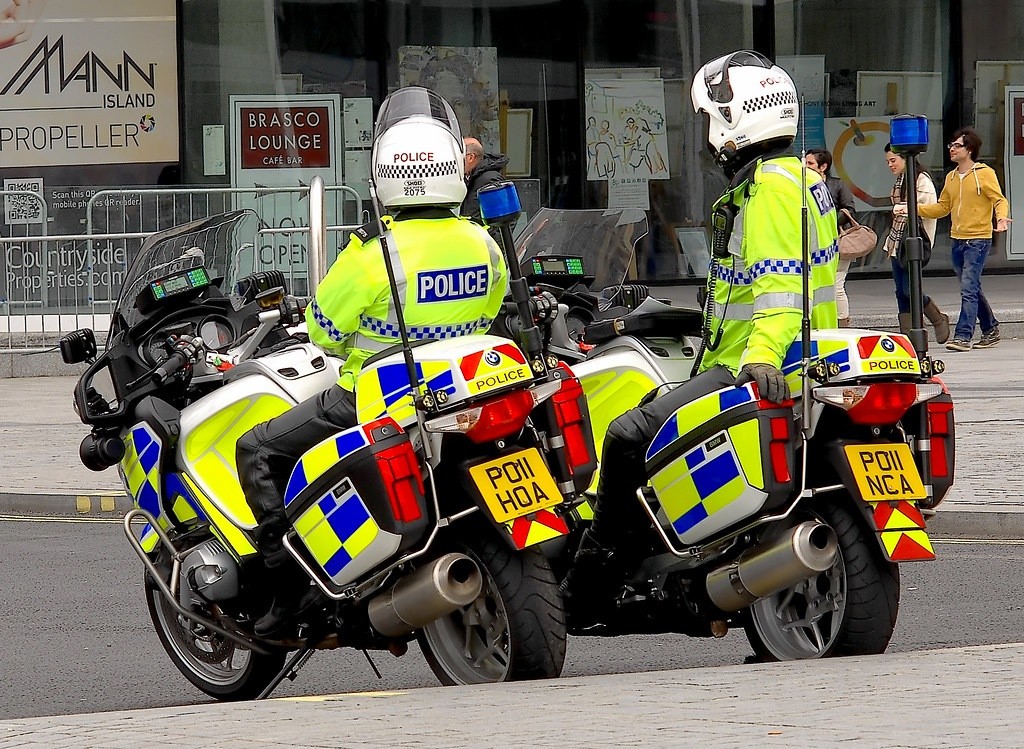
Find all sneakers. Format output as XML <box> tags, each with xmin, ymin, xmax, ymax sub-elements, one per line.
<box><xmin>946</xmin><ymin>341</ymin><xmax>971</xmax><ymax>351</ymax></box>
<box><xmin>972</xmin><ymin>331</ymin><xmax>1001</xmax><ymax>348</ymax></box>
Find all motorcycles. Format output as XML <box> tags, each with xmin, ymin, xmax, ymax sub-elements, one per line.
<box><xmin>486</xmin><ymin>112</ymin><xmax>956</xmax><ymax>663</ymax></box>
<box><xmin>59</xmin><ymin>178</ymin><xmax>599</xmax><ymax>701</ymax></box>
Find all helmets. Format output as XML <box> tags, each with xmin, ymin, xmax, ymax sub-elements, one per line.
<box><xmin>372</xmin><ymin>86</ymin><xmax>469</xmax><ymax>209</ymax></box>
<box><xmin>692</xmin><ymin>50</ymin><xmax>801</xmax><ymax>163</ymax></box>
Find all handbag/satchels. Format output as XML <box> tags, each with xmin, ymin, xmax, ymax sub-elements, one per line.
<box><xmin>837</xmin><ymin>209</ymin><xmax>877</xmax><ymax>260</ymax></box>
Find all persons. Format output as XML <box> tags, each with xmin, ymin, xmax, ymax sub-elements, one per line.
<box><xmin>453</xmin><ymin>132</ymin><xmax>520</xmax><ymax>266</ymax></box>
<box><xmin>802</xmin><ymin>145</ymin><xmax>857</xmax><ymax>330</ymax></box>
<box><xmin>236</xmin><ymin>85</ymin><xmax>509</xmax><ymax>639</ymax></box>
<box><xmin>555</xmin><ymin>51</ymin><xmax>841</xmax><ymax>625</ymax></box>
<box><xmin>893</xmin><ymin>112</ymin><xmax>1012</xmax><ymax>353</ymax></box>
<box><xmin>880</xmin><ymin>140</ymin><xmax>950</xmax><ymax>346</ymax></box>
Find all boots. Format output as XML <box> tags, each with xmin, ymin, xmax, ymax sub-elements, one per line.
<box><xmin>253</xmin><ymin>520</ymin><xmax>322</xmax><ymax>635</ymax></box>
<box><xmin>924</xmin><ymin>296</ymin><xmax>950</xmax><ymax>344</ymax></box>
<box><xmin>558</xmin><ymin>528</ymin><xmax>618</xmax><ymax>621</ymax></box>
<box><xmin>897</xmin><ymin>313</ymin><xmax>912</xmax><ymax>333</ymax></box>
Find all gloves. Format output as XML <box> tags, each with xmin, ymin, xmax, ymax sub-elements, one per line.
<box><xmin>735</xmin><ymin>365</ymin><xmax>790</xmax><ymax>404</ymax></box>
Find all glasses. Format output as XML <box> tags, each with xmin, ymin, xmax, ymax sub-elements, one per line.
<box><xmin>948</xmin><ymin>143</ymin><xmax>964</xmax><ymax>149</ymax></box>
